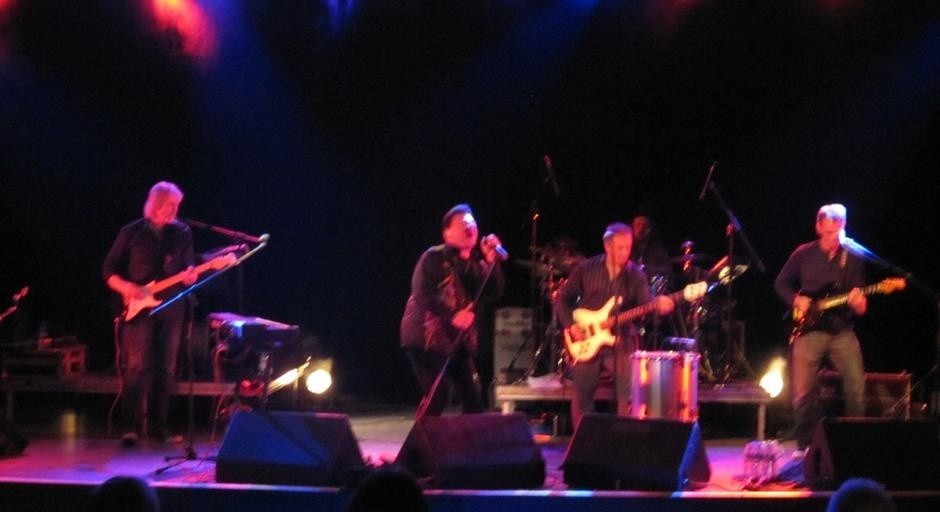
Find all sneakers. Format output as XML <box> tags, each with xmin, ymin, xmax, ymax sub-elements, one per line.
<box><xmin>163</xmin><ymin>434</ymin><xmax>183</xmax><ymax>443</ymax></box>
<box><xmin>120</xmin><ymin>432</ymin><xmax>138</xmax><ymax>448</ymax></box>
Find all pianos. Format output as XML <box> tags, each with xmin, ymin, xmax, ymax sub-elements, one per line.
<box><xmin>208</xmin><ymin>312</ymin><xmax>299</xmax><ymax>351</ymax></box>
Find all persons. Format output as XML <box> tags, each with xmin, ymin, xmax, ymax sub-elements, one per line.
<box><xmin>554</xmin><ymin>222</ymin><xmax>674</xmax><ymax>431</ymax></box>
<box><xmin>100</xmin><ymin>181</ymin><xmax>199</xmax><ymax>445</ymax></box>
<box><xmin>630</xmin><ymin>214</ymin><xmax>673</xmax><ymax>290</ymax></box>
<box><xmin>774</xmin><ymin>202</ymin><xmax>868</xmax><ymax>450</ymax></box>
<box><xmin>398</xmin><ymin>202</ymin><xmax>507</xmax><ymax>418</ymax></box>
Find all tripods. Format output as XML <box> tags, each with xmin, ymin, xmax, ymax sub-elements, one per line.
<box><xmin>515</xmin><ymin>293</ymin><xmax>568</xmax><ymax>389</ymax></box>
<box><xmin>154</xmin><ymin>299</ymin><xmax>218</xmax><ymax>476</ymax></box>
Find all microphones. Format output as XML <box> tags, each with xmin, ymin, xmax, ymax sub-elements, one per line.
<box><xmin>692</xmin><ymin>157</ymin><xmax>724</xmax><ymax>200</ymax></box>
<box><xmin>542</xmin><ymin>152</ymin><xmax>559</xmax><ymax>191</ymax></box>
<box><xmin>837</xmin><ymin>227</ymin><xmax>867</xmax><ymax>260</ymax></box>
<box><xmin>481</xmin><ymin>232</ymin><xmax>508</xmax><ymax>259</ymax></box>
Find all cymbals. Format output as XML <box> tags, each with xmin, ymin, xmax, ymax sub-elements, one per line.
<box><xmin>671</xmin><ymin>255</ymin><xmax>713</xmax><ymax>263</ymax></box>
<box><xmin>718</xmin><ymin>264</ymin><xmax>748</xmax><ymax>285</ymax></box>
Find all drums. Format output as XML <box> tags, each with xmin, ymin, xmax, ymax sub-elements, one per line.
<box><xmin>629</xmin><ymin>351</ymin><xmax>698</xmax><ymax>422</ymax></box>
<box><xmin>650</xmin><ymin>276</ymin><xmax>668</xmax><ymax>298</ymax></box>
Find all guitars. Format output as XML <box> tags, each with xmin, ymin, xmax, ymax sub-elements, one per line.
<box><xmin>789</xmin><ymin>277</ymin><xmax>905</xmax><ymax>343</ymax></box>
<box><xmin>564</xmin><ymin>281</ymin><xmax>708</xmax><ymax>362</ymax></box>
<box><xmin>122</xmin><ymin>253</ymin><xmax>232</xmax><ymax>321</ymax></box>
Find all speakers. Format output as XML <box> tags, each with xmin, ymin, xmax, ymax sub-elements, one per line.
<box><xmin>390</xmin><ymin>411</ymin><xmax>546</xmax><ymax>491</ymax></box>
<box><xmin>801</xmin><ymin>414</ymin><xmax>935</xmax><ymax>486</ymax></box>
<box><xmin>559</xmin><ymin>411</ymin><xmax>714</xmax><ymax>490</ymax></box>
<box><xmin>214</xmin><ymin>407</ymin><xmax>369</xmax><ymax>489</ymax></box>
